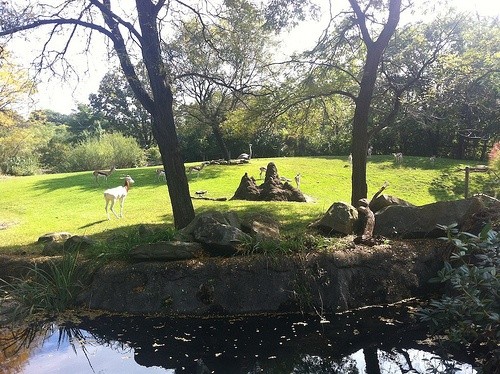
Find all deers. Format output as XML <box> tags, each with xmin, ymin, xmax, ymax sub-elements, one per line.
<box><xmin>188</xmin><ymin>163</ymin><xmax>205</xmax><ymax>180</ymax></box>
<box><xmin>104</xmin><ymin>174</ymin><xmax>135</xmax><ymax>221</ymax></box>
<box><xmin>93</xmin><ymin>165</ymin><xmax>116</xmax><ymax>187</ymax></box>
<box><xmin>155</xmin><ymin>169</ymin><xmax>166</xmax><ymax>182</ymax></box>
